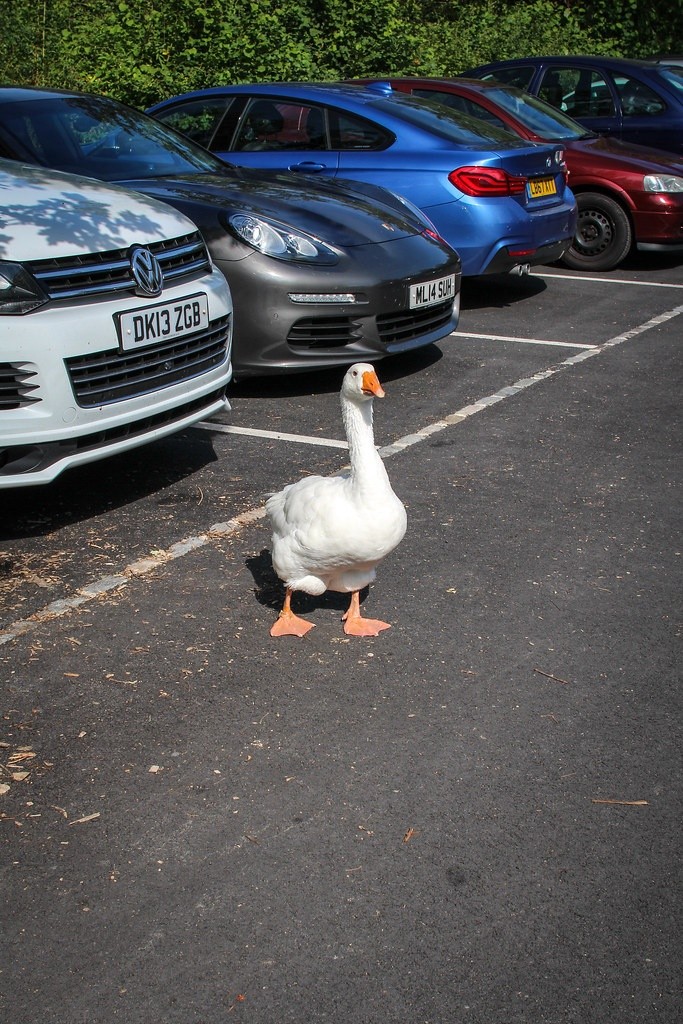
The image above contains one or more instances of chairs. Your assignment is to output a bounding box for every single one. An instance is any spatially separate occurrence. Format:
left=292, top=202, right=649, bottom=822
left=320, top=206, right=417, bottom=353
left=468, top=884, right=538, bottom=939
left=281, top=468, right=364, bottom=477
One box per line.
left=241, top=103, right=293, bottom=151
left=544, top=73, right=562, bottom=107
left=305, top=108, right=326, bottom=149
left=565, top=79, right=591, bottom=116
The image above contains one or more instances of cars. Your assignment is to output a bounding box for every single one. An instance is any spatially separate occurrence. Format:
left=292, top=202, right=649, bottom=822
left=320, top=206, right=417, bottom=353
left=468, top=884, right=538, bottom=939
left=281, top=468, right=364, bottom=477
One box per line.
left=455, top=54, right=683, bottom=156
left=0, top=153, right=236, bottom=496
left=65, top=84, right=579, bottom=290
left=561, top=58, right=683, bottom=115
left=257, top=76, right=683, bottom=271
left=0, top=85, right=464, bottom=379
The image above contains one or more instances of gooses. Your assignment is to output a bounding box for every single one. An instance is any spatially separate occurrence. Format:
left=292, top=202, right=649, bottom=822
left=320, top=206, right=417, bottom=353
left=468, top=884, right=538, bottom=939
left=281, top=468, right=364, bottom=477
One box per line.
left=262, top=363, right=409, bottom=637
